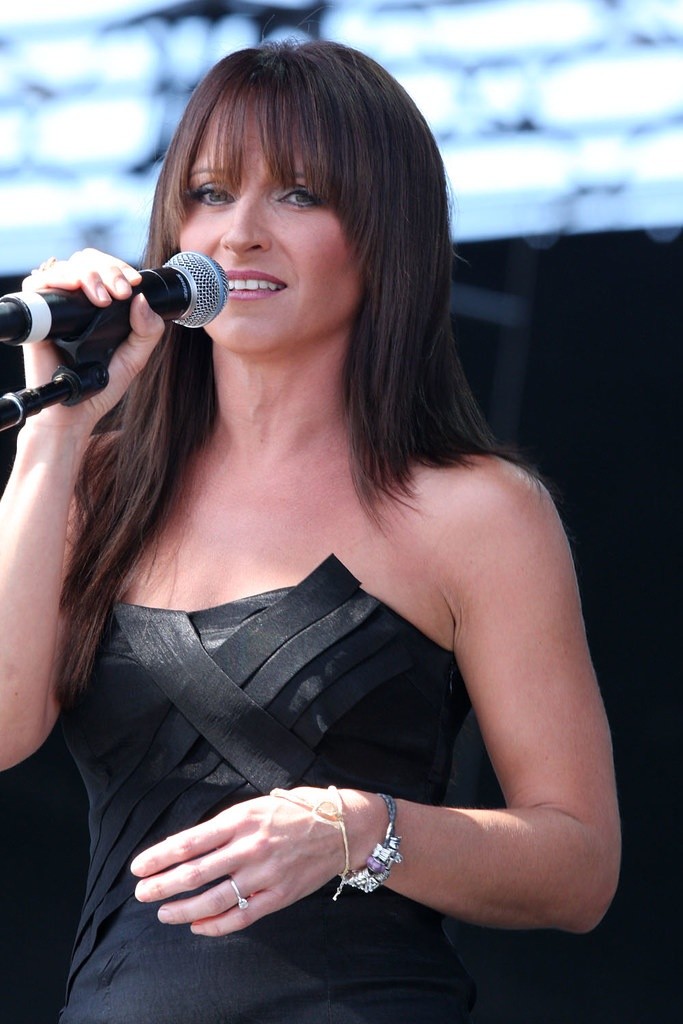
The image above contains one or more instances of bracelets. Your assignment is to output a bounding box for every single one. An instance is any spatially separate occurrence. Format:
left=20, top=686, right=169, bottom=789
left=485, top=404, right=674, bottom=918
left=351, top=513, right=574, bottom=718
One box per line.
left=270, top=784, right=350, bottom=876
left=337, top=794, right=403, bottom=892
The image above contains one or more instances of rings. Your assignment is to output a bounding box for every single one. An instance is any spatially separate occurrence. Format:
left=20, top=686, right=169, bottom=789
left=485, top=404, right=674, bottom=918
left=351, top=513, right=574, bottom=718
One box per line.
left=228, top=878, right=250, bottom=910
left=36, top=256, right=56, bottom=272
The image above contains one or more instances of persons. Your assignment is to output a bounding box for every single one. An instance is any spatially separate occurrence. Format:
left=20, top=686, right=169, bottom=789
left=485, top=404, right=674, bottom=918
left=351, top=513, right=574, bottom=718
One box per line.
left=0, top=42, right=619, bottom=1024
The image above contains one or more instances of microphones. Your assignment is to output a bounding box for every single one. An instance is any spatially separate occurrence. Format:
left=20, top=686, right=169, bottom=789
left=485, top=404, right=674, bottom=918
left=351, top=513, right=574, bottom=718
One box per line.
left=0, top=252, right=230, bottom=348
